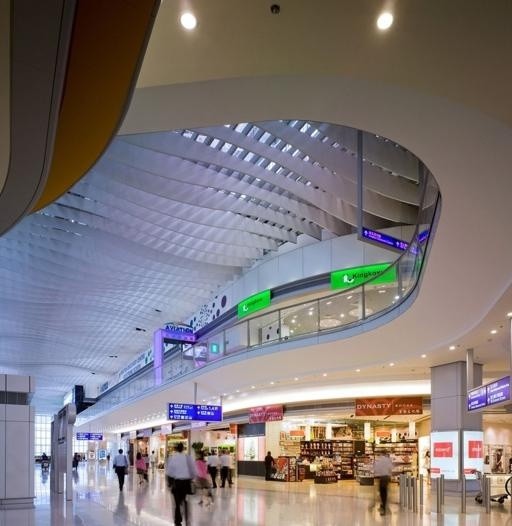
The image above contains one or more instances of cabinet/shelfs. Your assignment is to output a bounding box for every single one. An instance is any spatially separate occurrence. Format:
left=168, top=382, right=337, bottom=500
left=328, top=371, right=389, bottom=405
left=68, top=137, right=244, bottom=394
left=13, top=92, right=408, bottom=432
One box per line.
left=298, top=437, right=418, bottom=485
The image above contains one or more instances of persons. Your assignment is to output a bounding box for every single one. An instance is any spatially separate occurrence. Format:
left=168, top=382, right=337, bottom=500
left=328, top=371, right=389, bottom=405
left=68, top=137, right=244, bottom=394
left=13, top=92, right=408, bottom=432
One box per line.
left=423, top=450, right=432, bottom=486
left=264, top=450, right=276, bottom=481
left=41, top=452, right=49, bottom=470
left=112, top=449, right=156, bottom=492
left=475, top=446, right=504, bottom=504
left=372, top=448, right=394, bottom=517
left=165, top=440, right=235, bottom=526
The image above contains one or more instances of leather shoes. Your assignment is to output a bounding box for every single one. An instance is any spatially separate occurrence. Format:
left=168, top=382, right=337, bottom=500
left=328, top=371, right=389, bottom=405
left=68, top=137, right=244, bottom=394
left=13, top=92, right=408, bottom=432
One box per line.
left=212, top=482, right=235, bottom=488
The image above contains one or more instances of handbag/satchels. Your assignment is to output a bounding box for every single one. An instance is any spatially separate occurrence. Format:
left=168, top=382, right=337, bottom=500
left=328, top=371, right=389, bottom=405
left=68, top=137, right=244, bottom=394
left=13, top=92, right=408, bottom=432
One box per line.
left=189, top=482, right=197, bottom=495
